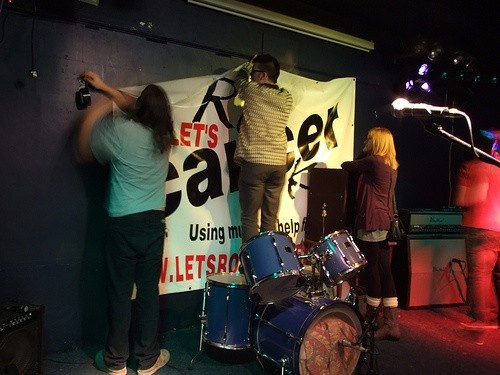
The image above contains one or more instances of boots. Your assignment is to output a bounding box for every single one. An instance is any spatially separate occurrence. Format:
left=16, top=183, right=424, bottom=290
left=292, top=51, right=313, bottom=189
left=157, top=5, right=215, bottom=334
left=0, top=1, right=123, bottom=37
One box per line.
left=367, top=307, right=400, bottom=342
left=362, top=303, right=383, bottom=330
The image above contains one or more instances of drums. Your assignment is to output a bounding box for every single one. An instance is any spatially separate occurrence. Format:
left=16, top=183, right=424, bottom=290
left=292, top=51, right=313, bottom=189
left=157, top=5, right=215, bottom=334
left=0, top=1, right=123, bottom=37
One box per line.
left=249, top=286, right=368, bottom=375
left=205, top=271, right=259, bottom=352
left=238, top=230, right=308, bottom=305
left=306, top=228, right=369, bottom=288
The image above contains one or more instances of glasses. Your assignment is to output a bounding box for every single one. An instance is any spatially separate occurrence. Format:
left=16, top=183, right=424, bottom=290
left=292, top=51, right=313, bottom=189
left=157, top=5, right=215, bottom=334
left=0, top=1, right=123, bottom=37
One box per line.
left=252, top=69, right=267, bottom=73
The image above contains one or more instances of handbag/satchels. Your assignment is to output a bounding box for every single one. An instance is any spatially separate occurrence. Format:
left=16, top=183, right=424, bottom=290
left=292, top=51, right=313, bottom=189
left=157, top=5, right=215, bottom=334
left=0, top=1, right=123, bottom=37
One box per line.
left=390, top=214, right=405, bottom=242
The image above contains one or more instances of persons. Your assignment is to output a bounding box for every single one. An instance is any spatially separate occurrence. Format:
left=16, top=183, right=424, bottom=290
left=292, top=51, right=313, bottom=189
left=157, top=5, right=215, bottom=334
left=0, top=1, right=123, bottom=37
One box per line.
left=72, top=70, right=177, bottom=375
left=454, top=128, right=500, bottom=330
left=234, top=55, right=294, bottom=274
left=340, top=127, right=400, bottom=339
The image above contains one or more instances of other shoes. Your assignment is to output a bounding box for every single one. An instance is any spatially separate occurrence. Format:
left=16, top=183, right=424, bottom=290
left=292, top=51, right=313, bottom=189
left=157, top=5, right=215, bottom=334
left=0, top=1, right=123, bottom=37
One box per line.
left=137, top=349, right=171, bottom=375
left=96, top=349, right=127, bottom=375
left=460, top=318, right=499, bottom=329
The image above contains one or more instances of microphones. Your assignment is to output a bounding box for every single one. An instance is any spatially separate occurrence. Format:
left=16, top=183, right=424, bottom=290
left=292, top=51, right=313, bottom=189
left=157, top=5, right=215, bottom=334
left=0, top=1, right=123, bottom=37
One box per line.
left=452, top=258, right=464, bottom=263
left=338, top=338, right=368, bottom=353
left=390, top=98, right=465, bottom=120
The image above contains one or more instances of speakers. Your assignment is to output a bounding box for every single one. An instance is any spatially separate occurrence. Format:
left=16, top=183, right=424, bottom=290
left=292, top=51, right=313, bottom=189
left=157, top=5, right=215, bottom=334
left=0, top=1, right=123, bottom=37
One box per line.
left=391, top=232, right=469, bottom=310
left=0, top=306, right=43, bottom=375
